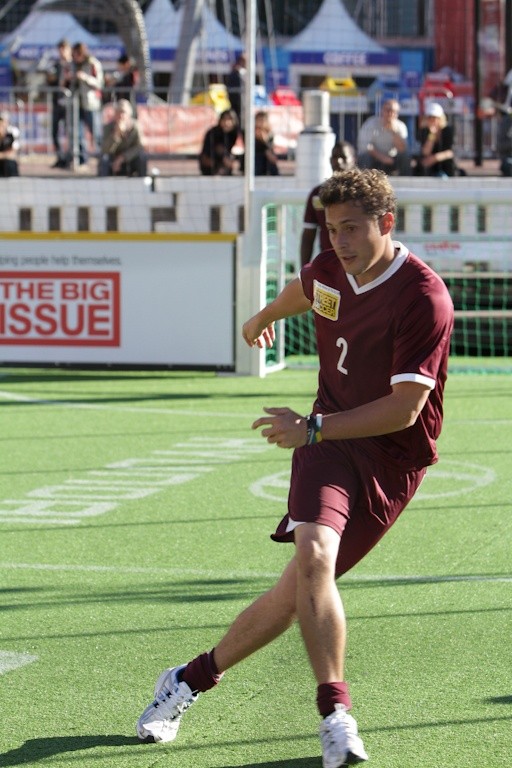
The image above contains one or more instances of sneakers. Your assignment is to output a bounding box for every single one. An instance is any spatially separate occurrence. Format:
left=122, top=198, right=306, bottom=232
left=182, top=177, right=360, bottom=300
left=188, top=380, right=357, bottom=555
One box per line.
left=135, top=663, right=199, bottom=743
left=317, top=704, right=369, bottom=768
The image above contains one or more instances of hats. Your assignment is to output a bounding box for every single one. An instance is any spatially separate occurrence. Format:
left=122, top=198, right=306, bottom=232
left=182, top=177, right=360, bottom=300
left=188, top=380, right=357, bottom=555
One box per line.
left=425, top=103, right=444, bottom=117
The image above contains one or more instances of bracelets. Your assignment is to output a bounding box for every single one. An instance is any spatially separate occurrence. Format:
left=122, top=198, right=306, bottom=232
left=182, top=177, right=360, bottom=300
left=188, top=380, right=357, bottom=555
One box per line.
left=304, top=412, right=323, bottom=445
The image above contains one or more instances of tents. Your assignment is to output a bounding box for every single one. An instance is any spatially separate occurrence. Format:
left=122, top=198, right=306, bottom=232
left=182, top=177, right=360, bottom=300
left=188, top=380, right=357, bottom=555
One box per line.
left=141, top=0, right=243, bottom=77
left=0, top=0, right=127, bottom=80
left=276, top=0, right=399, bottom=93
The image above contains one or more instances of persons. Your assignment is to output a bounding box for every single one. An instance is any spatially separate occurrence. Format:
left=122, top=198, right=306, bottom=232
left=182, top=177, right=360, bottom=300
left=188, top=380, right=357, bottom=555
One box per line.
left=137, top=164, right=457, bottom=768
left=355, top=98, right=410, bottom=177
left=0, top=110, right=23, bottom=176
left=198, top=110, right=298, bottom=176
left=96, top=98, right=146, bottom=178
left=409, top=101, right=455, bottom=175
left=301, top=140, right=358, bottom=271
left=43, top=40, right=104, bottom=168
